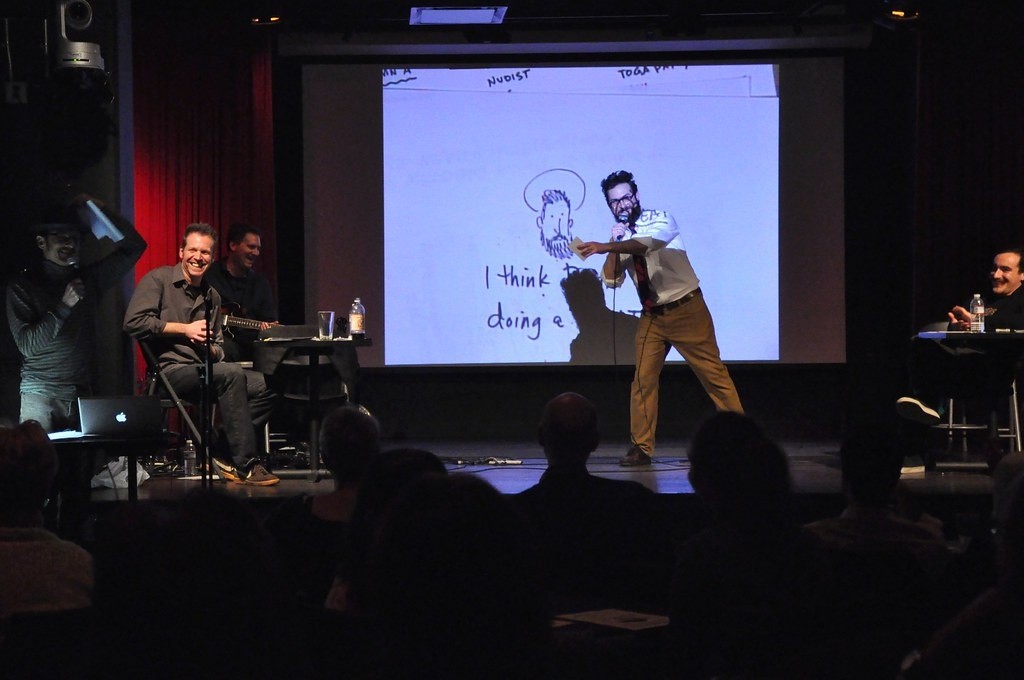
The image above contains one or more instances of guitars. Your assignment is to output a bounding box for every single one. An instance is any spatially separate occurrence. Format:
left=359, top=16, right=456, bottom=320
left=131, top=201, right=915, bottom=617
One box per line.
left=221, top=303, right=289, bottom=340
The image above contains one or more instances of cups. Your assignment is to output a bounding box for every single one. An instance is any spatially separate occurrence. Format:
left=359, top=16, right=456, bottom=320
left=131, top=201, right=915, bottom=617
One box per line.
left=318, top=311, right=335, bottom=341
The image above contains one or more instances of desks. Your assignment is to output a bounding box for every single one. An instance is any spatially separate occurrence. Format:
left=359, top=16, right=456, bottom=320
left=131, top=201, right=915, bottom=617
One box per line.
left=918, top=331, right=1024, bottom=472
left=254, top=337, right=372, bottom=483
left=48, top=436, right=156, bottom=528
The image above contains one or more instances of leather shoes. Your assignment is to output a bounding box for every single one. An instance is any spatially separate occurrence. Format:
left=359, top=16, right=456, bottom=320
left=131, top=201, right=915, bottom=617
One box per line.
left=620, top=446, right=652, bottom=465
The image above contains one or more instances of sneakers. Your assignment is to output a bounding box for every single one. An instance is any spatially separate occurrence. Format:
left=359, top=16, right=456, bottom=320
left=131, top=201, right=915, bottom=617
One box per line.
left=245, top=463, right=279, bottom=485
left=212, top=456, right=241, bottom=481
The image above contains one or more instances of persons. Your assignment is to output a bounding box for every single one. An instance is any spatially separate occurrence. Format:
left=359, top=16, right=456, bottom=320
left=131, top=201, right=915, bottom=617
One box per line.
left=893, top=241, right=1024, bottom=426
left=5, top=194, right=145, bottom=430
left=122, top=221, right=288, bottom=488
left=0, top=391, right=1024, bottom=680
left=577, top=169, right=748, bottom=466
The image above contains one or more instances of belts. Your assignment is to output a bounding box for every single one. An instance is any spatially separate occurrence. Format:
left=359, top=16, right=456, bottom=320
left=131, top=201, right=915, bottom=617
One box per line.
left=643, top=287, right=701, bottom=312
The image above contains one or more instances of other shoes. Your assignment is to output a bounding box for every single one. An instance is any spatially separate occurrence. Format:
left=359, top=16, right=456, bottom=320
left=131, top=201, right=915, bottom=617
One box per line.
left=898, top=457, right=926, bottom=474
left=895, top=396, right=942, bottom=428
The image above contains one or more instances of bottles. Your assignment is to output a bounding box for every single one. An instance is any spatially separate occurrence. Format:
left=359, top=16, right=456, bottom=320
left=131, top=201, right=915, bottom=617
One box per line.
left=970, top=293, right=985, bottom=333
left=349, top=298, right=365, bottom=339
left=183, top=440, right=196, bottom=475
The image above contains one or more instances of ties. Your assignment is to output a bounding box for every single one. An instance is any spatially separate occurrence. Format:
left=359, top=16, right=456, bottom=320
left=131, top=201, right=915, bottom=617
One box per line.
left=627, top=221, right=659, bottom=307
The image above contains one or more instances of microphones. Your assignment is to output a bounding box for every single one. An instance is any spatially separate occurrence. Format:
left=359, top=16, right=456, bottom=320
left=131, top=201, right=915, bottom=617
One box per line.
left=67, top=256, right=81, bottom=281
left=616, top=213, right=629, bottom=243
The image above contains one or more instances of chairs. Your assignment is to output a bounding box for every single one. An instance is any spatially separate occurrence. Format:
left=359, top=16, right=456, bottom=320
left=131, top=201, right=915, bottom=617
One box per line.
left=138, top=339, right=228, bottom=484
left=916, top=322, right=1016, bottom=457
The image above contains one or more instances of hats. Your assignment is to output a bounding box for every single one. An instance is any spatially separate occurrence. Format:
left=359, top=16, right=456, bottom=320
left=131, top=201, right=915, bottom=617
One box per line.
left=28, top=202, right=91, bottom=235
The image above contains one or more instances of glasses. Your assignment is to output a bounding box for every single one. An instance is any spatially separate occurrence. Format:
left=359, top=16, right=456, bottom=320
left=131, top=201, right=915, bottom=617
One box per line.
left=44, top=229, right=82, bottom=246
left=986, top=520, right=1009, bottom=536
left=609, top=191, right=635, bottom=209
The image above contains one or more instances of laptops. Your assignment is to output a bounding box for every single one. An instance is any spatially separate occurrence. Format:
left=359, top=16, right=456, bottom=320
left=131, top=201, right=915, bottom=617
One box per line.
left=77, top=396, right=163, bottom=436
